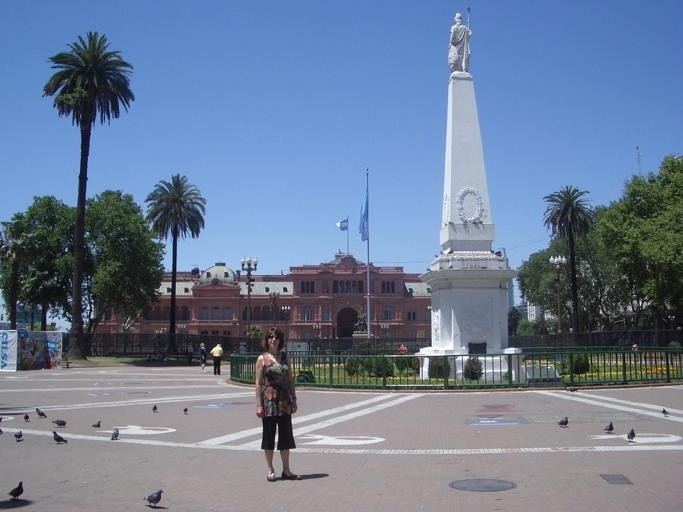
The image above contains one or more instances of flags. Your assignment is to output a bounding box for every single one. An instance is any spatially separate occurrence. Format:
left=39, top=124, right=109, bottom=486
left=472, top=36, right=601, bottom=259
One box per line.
left=335, top=216, right=347, bottom=231
left=358, top=180, right=368, bottom=241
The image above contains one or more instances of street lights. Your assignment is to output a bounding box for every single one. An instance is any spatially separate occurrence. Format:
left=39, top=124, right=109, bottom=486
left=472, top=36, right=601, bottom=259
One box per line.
left=269, top=288, right=280, bottom=324
left=312, top=324, right=322, bottom=337
left=240, top=256, right=259, bottom=337
left=381, top=324, right=390, bottom=346
left=549, top=256, right=566, bottom=333
left=281, top=305, right=291, bottom=353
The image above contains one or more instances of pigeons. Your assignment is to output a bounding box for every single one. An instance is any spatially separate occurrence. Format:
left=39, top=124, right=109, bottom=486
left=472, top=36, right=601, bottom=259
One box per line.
left=661, top=407, right=670, bottom=417
left=603, top=422, right=614, bottom=433
left=14, top=407, right=68, bottom=444
left=92, top=420, right=101, bottom=427
left=626, top=428, right=636, bottom=441
left=142, top=487, right=164, bottom=507
left=183, top=408, right=189, bottom=415
left=111, top=427, right=120, bottom=440
left=6, top=480, right=24, bottom=500
left=556, top=416, right=569, bottom=429
left=152, top=404, right=157, bottom=412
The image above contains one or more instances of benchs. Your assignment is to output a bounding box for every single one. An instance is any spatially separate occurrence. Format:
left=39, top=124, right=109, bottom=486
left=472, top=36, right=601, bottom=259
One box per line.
left=61, top=361, right=72, bottom=368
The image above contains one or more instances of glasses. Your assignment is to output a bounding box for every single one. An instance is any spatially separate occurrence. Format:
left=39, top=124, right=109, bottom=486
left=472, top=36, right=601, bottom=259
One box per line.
left=269, top=335, right=279, bottom=339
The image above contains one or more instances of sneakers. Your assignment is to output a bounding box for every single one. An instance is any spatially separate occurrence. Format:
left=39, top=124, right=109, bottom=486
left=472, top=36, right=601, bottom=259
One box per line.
left=281, top=471, right=303, bottom=480
left=213, top=372, right=219, bottom=374
left=267, top=471, right=276, bottom=480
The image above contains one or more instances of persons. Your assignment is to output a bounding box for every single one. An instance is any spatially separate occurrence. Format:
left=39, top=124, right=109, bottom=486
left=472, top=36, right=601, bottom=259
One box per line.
left=210, top=343, right=223, bottom=374
left=187, top=341, right=194, bottom=365
left=199, top=342, right=206, bottom=372
left=632, top=341, right=638, bottom=361
left=447, top=12, right=472, bottom=72
left=255, top=326, right=300, bottom=481
